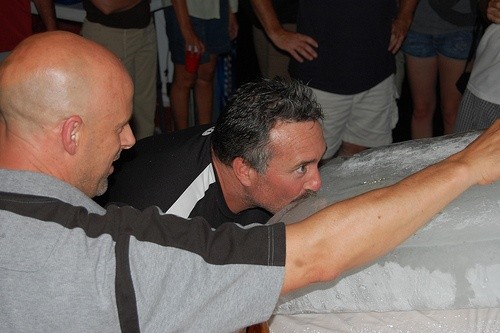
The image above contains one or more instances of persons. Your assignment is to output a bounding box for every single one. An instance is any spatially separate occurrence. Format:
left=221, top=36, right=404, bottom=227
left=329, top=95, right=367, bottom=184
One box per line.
left=454, top=0, right=500, bottom=136
left=161, top=0, right=240, bottom=137
left=92, top=72, right=327, bottom=230
left=250, top=0, right=420, bottom=164
left=244, top=0, right=299, bottom=81
left=399, top=0, right=477, bottom=139
left=0, top=0, right=57, bottom=63
left=80, top=0, right=158, bottom=140
left=0, top=30, right=500, bottom=333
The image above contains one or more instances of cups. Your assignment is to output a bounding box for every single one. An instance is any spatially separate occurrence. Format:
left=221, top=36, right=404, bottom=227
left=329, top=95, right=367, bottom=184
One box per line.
left=185, top=45, right=201, bottom=71
